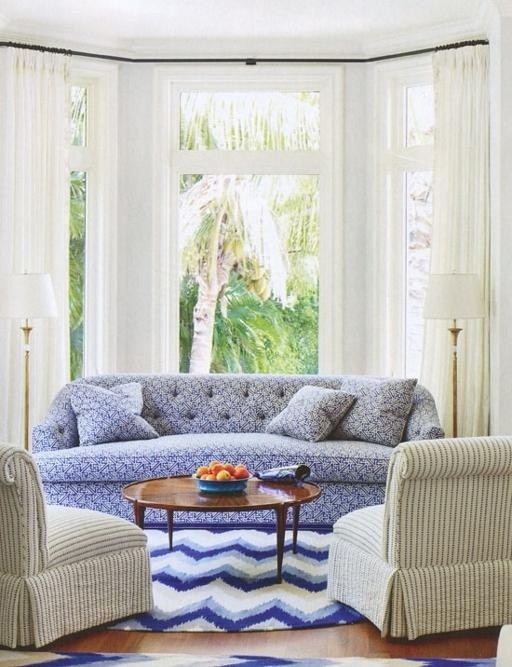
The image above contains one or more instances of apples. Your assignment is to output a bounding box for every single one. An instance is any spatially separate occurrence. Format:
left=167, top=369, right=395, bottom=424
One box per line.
left=196, top=461, right=250, bottom=480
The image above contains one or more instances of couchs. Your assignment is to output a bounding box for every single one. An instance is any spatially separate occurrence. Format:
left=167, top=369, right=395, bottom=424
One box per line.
left=29, top=372, right=445, bottom=525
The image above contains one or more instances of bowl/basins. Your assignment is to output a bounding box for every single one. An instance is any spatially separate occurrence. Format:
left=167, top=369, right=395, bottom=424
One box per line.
left=192, top=472, right=252, bottom=494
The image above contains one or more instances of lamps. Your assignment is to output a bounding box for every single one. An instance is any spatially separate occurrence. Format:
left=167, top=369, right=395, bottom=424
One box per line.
left=425, top=271, right=486, bottom=435
left=9, top=268, right=59, bottom=449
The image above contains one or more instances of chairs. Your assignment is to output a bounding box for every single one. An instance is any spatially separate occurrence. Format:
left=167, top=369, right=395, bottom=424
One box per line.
left=0, top=440, right=157, bottom=648
left=323, top=432, right=512, bottom=642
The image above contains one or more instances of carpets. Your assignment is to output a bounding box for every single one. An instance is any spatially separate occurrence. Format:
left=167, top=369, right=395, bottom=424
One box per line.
left=97, top=515, right=370, bottom=633
left=1, top=652, right=500, bottom=665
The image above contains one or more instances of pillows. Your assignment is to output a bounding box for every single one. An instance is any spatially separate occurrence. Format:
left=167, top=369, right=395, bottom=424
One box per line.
left=333, top=376, right=416, bottom=447
left=264, top=382, right=358, bottom=441
left=61, top=380, right=160, bottom=446
